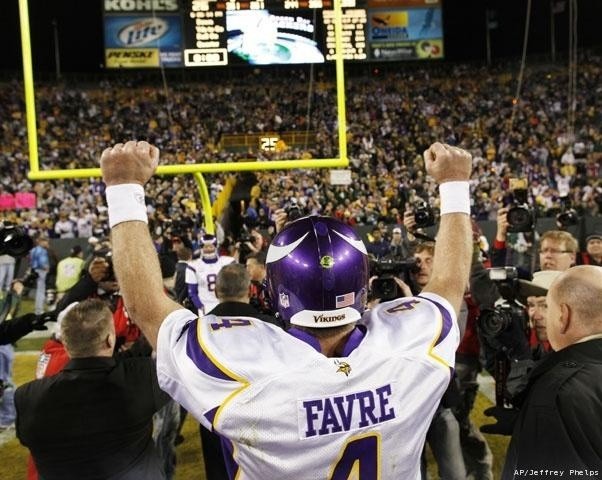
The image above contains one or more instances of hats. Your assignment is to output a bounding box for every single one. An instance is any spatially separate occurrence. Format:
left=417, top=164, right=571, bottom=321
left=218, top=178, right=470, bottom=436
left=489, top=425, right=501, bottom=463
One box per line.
left=392, top=227, right=402, bottom=234
left=70, top=245, right=82, bottom=257
left=512, top=270, right=565, bottom=299
left=584, top=230, right=602, bottom=242
left=87, top=235, right=99, bottom=244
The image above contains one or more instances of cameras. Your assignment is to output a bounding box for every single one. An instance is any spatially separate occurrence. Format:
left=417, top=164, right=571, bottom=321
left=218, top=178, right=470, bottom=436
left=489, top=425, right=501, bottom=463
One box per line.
left=506, top=207, right=533, bottom=231
left=0, top=220, right=35, bottom=258
left=409, top=201, right=440, bottom=227
left=100, top=255, right=115, bottom=281
left=558, top=211, right=577, bottom=226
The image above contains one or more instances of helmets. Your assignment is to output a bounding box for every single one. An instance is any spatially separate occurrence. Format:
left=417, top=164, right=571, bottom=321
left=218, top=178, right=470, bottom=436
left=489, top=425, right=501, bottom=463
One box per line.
left=263, top=214, right=370, bottom=329
left=199, top=234, right=219, bottom=260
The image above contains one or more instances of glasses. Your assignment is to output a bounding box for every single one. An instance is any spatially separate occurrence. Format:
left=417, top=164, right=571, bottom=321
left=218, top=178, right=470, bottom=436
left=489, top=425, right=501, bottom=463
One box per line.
left=538, top=246, right=573, bottom=256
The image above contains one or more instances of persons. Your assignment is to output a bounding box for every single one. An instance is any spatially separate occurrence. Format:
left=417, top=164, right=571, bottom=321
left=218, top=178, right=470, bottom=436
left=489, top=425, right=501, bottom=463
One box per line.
left=0, top=71, right=200, bottom=479
left=98, top=138, right=478, bottom=480
left=424, top=48, right=599, bottom=479
left=200, top=60, right=425, bottom=314
